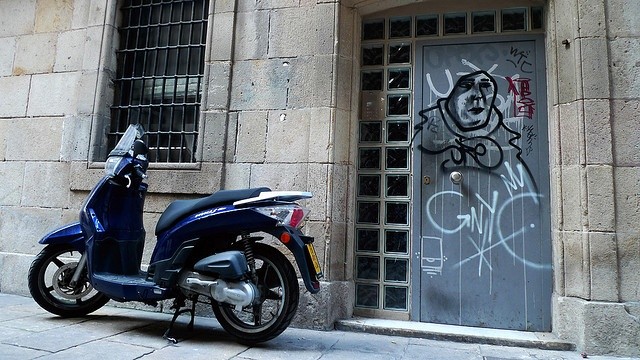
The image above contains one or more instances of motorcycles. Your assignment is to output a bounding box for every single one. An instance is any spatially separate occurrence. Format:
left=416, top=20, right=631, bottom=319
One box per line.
left=28, top=123, right=323, bottom=345
